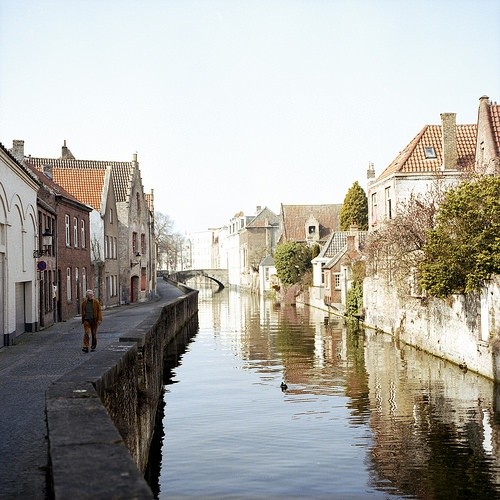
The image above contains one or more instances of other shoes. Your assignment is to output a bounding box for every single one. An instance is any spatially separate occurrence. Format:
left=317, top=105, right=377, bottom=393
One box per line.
left=82, top=347, right=89, bottom=352
left=91, top=345, right=96, bottom=350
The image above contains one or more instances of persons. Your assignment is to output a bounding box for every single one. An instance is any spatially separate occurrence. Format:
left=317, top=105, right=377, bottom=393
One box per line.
left=81, top=290, right=103, bottom=352
